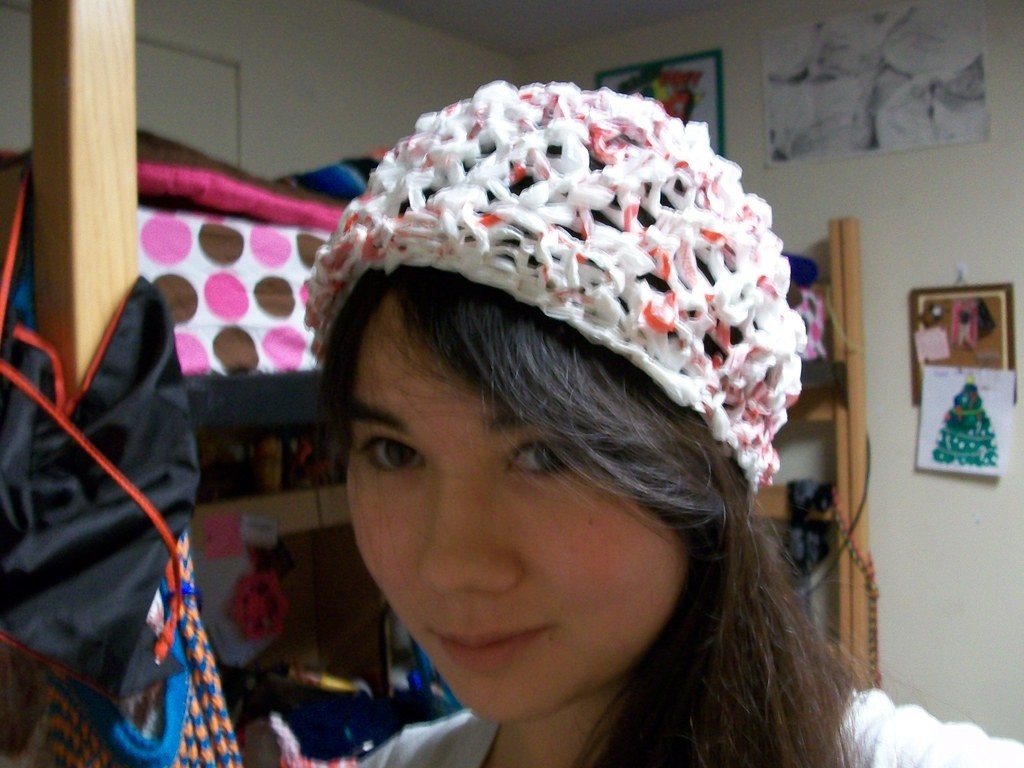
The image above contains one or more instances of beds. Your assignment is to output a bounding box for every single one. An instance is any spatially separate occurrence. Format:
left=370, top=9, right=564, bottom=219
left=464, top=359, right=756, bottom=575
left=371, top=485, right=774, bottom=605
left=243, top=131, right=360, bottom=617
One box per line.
left=0, top=0, right=867, bottom=429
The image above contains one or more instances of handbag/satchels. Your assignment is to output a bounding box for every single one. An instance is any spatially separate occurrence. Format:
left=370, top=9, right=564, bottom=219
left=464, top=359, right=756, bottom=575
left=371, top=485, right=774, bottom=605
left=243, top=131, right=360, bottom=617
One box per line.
left=40, top=529, right=244, bottom=768
left=0, top=359, right=244, bottom=768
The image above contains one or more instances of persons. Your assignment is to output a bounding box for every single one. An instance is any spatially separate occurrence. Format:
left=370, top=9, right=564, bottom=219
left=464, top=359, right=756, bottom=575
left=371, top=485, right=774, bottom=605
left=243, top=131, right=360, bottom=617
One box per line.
left=305, top=78, right=1024, bottom=768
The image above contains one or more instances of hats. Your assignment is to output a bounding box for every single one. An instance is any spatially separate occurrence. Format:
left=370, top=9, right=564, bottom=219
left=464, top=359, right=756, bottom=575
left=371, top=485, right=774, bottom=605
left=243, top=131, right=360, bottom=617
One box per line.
left=304, top=81, right=808, bottom=494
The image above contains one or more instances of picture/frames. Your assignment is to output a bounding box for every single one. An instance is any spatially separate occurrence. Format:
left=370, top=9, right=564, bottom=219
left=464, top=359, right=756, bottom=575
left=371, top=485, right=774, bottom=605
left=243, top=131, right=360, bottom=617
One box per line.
left=908, top=283, right=1014, bottom=408
left=594, top=48, right=724, bottom=159
